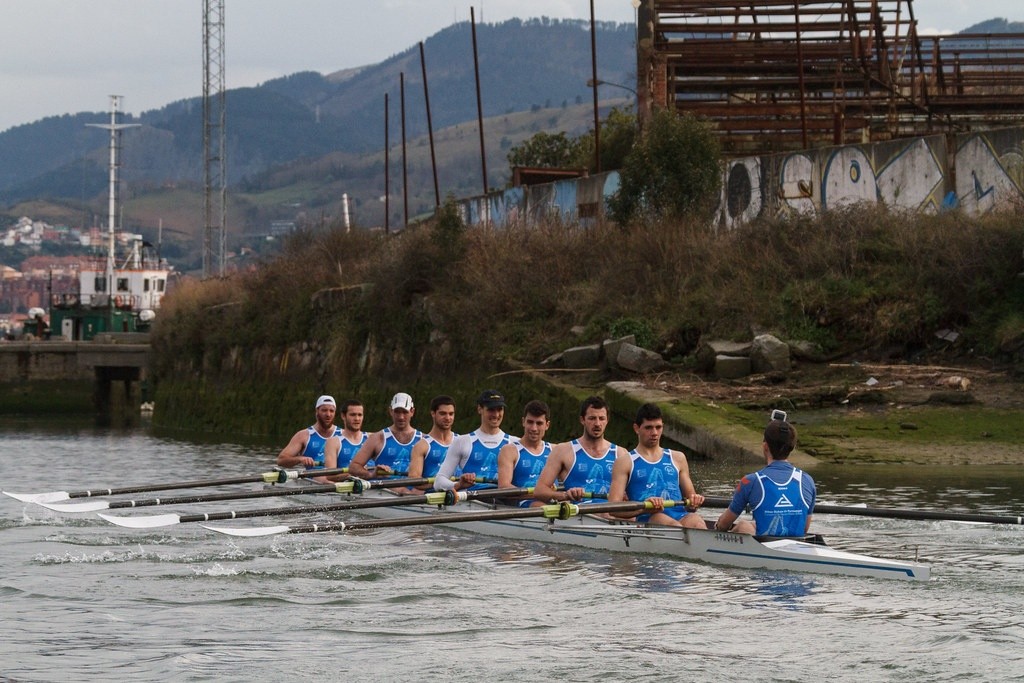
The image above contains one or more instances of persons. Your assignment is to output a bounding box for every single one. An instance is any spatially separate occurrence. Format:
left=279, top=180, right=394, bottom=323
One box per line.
left=608, top=403, right=708, bottom=529
left=325, top=399, right=375, bottom=482
left=277, top=395, right=345, bottom=482
left=434, top=389, right=522, bottom=492
left=498, top=400, right=558, bottom=508
left=408, top=395, right=460, bottom=493
left=534, top=397, right=636, bottom=522
left=348, top=393, right=428, bottom=496
left=714, top=420, right=816, bottom=536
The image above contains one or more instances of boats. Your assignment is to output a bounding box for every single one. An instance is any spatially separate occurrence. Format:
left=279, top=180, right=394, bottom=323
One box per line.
left=261, top=465, right=937, bottom=587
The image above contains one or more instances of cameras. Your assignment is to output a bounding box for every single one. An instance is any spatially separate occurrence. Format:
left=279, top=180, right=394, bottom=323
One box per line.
left=771, top=409, right=786, bottom=424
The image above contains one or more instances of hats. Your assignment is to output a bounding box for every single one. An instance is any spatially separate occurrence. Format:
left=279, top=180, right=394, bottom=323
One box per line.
left=391, top=393, right=413, bottom=411
left=478, top=390, right=507, bottom=409
left=316, top=395, right=336, bottom=408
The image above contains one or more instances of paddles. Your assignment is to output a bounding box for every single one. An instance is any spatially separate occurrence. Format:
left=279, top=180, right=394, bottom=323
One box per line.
left=1, top=463, right=377, bottom=504
left=581, top=492, right=610, bottom=499
left=683, top=496, right=1024, bottom=527
left=197, top=498, right=692, bottom=539
left=92, top=482, right=564, bottom=519
left=36, top=474, right=461, bottom=514
left=449, top=475, right=498, bottom=485
left=377, top=469, right=409, bottom=476
